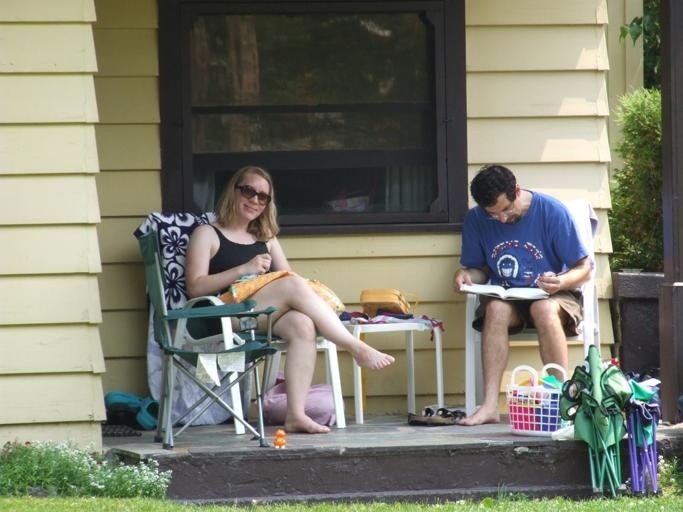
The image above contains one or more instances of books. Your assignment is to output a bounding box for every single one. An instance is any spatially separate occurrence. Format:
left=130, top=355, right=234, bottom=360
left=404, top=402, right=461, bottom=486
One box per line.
left=459, top=282, right=551, bottom=301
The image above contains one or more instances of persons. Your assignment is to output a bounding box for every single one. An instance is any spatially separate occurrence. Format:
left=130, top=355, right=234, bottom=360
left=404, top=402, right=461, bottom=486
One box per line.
left=183, top=164, right=394, bottom=432
left=452, top=163, right=594, bottom=427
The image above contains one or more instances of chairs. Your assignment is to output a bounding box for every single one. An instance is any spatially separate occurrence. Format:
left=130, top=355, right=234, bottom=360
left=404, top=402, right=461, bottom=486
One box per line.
left=463, top=201, right=600, bottom=419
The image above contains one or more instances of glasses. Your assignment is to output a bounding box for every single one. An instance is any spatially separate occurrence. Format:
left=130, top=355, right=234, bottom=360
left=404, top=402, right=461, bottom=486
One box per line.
left=236, top=185, right=271, bottom=205
left=482, top=201, right=515, bottom=221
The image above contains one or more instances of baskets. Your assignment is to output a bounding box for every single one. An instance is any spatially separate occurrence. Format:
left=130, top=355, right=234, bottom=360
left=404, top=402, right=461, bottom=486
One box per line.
left=506, top=363, right=573, bottom=437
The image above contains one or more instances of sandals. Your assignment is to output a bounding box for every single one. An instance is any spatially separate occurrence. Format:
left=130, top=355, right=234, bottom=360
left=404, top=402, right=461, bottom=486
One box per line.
left=104, top=391, right=159, bottom=430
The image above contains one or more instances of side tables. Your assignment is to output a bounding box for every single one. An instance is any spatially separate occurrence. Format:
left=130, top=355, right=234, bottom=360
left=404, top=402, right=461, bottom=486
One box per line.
left=341, top=316, right=445, bottom=425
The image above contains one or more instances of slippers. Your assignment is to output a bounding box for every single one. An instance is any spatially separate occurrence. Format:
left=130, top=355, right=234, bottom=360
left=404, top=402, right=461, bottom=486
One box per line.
left=409, top=408, right=465, bottom=426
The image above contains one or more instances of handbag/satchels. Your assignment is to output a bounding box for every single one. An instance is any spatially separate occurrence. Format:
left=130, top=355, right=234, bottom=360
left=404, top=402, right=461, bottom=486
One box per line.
left=360, top=287, right=420, bottom=316
